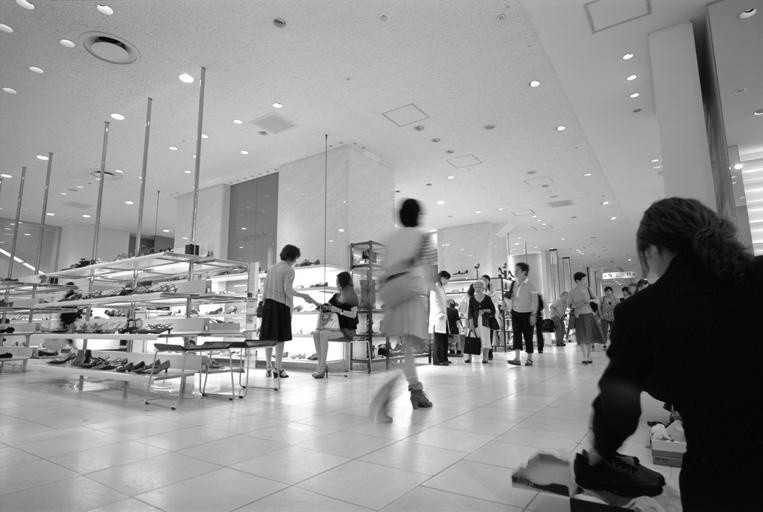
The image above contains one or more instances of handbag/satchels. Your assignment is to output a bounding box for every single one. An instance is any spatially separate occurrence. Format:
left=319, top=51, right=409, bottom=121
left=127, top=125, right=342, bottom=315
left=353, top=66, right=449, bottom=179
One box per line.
left=500, top=292, right=512, bottom=312
left=590, top=297, right=597, bottom=312
left=378, top=257, right=427, bottom=310
left=316, top=312, right=340, bottom=332
left=464, top=330, right=481, bottom=355
left=256, top=301, right=264, bottom=317
left=482, top=312, right=499, bottom=330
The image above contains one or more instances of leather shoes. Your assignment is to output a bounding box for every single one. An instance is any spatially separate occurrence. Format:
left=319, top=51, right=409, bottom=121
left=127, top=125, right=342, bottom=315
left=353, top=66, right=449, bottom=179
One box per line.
left=272, top=352, right=318, bottom=360
left=294, top=259, right=320, bottom=267
left=209, top=306, right=237, bottom=314
left=38, top=351, right=170, bottom=374
left=310, top=282, right=328, bottom=288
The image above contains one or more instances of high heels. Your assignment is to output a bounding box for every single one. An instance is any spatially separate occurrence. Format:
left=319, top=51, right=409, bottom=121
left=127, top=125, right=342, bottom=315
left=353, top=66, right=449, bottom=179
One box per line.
left=408, top=383, right=432, bottom=409
left=266, top=366, right=288, bottom=378
left=312, top=365, right=328, bottom=378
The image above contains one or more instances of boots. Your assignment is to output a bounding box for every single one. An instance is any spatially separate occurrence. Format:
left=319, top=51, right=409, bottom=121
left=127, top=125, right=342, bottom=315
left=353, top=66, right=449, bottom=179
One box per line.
left=358, top=280, right=375, bottom=309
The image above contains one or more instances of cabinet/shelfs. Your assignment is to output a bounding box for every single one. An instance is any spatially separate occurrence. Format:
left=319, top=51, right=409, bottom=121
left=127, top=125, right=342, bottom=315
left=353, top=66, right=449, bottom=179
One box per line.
left=0, top=251, right=519, bottom=411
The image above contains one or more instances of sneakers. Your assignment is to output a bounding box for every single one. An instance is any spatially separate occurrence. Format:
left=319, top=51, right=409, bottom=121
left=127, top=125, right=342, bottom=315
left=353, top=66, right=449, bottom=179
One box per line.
left=557, top=343, right=565, bottom=346
left=581, top=345, right=606, bottom=365
left=574, top=449, right=665, bottom=498
left=433, top=361, right=451, bottom=365
left=539, top=348, right=543, bottom=353
left=508, top=358, right=533, bottom=366
left=465, top=350, right=493, bottom=363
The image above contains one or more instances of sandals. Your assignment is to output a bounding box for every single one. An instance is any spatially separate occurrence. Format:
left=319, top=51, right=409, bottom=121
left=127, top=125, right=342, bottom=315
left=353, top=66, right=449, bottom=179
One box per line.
left=118, top=319, right=173, bottom=334
left=58, top=284, right=177, bottom=302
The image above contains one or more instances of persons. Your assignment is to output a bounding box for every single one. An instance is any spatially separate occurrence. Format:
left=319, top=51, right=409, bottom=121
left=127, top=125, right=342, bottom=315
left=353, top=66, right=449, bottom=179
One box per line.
left=313, top=272, right=358, bottom=378
left=60, top=283, right=77, bottom=346
left=428, top=263, right=648, bottom=366
left=258, top=244, right=310, bottom=377
left=589, top=198, right=763, bottom=512
left=367, top=199, right=437, bottom=422
